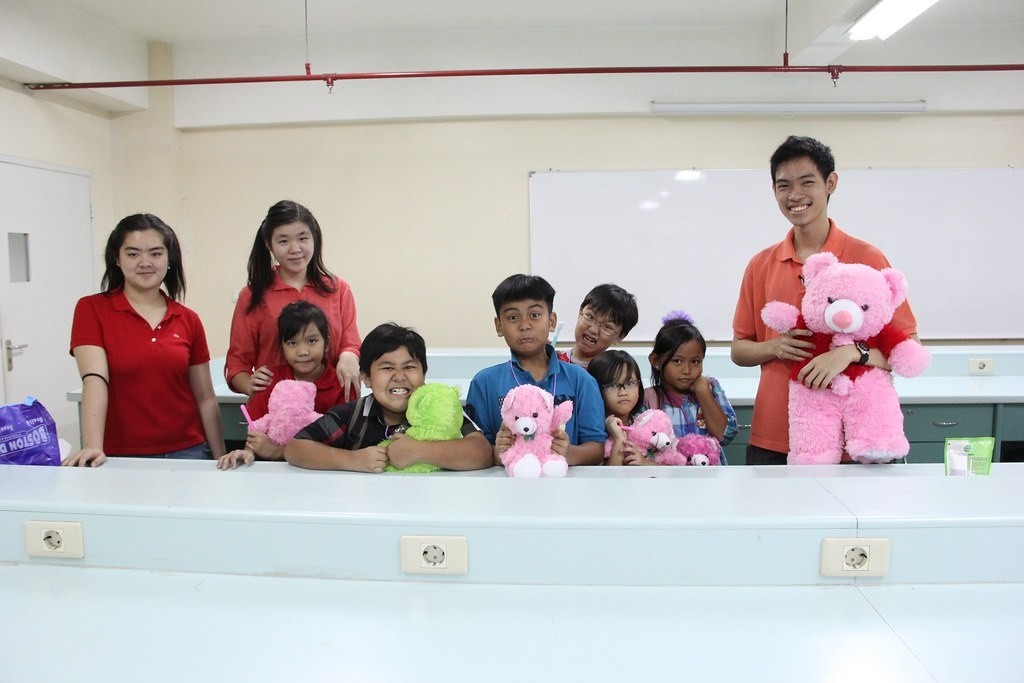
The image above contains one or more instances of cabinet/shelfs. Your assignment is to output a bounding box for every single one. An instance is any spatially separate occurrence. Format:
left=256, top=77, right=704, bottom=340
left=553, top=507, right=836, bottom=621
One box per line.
left=219, top=402, right=249, bottom=442
left=720, top=405, right=754, bottom=465
left=892, top=403, right=1004, bottom=463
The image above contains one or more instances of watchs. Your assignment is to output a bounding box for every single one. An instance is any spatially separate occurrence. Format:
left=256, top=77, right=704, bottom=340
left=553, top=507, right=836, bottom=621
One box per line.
left=856, top=340, right=870, bottom=366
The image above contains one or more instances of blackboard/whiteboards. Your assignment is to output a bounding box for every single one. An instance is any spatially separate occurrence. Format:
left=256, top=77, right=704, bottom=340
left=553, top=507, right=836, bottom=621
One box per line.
left=527, top=169, right=1023, bottom=347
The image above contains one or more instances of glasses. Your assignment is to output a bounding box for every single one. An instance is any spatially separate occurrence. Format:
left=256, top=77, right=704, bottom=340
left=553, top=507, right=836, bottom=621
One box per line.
left=602, top=379, right=640, bottom=393
left=580, top=312, right=623, bottom=337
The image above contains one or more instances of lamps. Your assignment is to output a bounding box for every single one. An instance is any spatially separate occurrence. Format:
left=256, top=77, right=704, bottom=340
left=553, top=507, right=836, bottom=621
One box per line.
left=648, top=100, right=928, bottom=116
left=837, top=0, right=941, bottom=42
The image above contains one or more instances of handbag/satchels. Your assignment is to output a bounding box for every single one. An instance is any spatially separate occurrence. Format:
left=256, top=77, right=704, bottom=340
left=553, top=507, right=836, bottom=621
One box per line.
left=1, top=395, right=61, bottom=466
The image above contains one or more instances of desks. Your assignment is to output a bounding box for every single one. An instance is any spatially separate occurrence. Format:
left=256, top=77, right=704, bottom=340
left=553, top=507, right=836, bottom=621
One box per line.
left=58, top=372, right=1024, bottom=404
left=0, top=561, right=1024, bottom=683
left=0, top=455, right=1024, bottom=520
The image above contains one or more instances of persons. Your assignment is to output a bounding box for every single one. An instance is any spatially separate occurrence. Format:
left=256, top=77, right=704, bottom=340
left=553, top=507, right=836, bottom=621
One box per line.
left=731, top=134, right=922, bottom=465
left=216, top=200, right=739, bottom=473
left=60, top=214, right=227, bottom=468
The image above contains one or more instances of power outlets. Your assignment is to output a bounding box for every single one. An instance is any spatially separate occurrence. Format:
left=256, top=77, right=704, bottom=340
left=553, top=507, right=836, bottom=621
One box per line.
left=968, top=357, right=998, bottom=374
left=399, top=535, right=469, bottom=575
left=24, top=521, right=85, bottom=560
left=819, top=537, right=890, bottom=578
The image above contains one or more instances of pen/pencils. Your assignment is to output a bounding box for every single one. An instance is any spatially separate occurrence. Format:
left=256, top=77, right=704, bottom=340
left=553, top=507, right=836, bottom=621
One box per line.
left=550, top=322, right=563, bottom=348
left=798, top=275, right=804, bottom=284
left=241, top=404, right=255, bottom=431
left=619, top=425, right=632, bottom=431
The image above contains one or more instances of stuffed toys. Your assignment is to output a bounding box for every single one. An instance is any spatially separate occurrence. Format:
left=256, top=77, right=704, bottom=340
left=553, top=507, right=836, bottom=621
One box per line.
left=603, top=407, right=686, bottom=466
left=248, top=379, right=326, bottom=446
left=673, top=431, right=721, bottom=467
left=500, top=384, right=574, bottom=476
left=379, top=384, right=464, bottom=473
left=759, top=252, right=930, bottom=465
